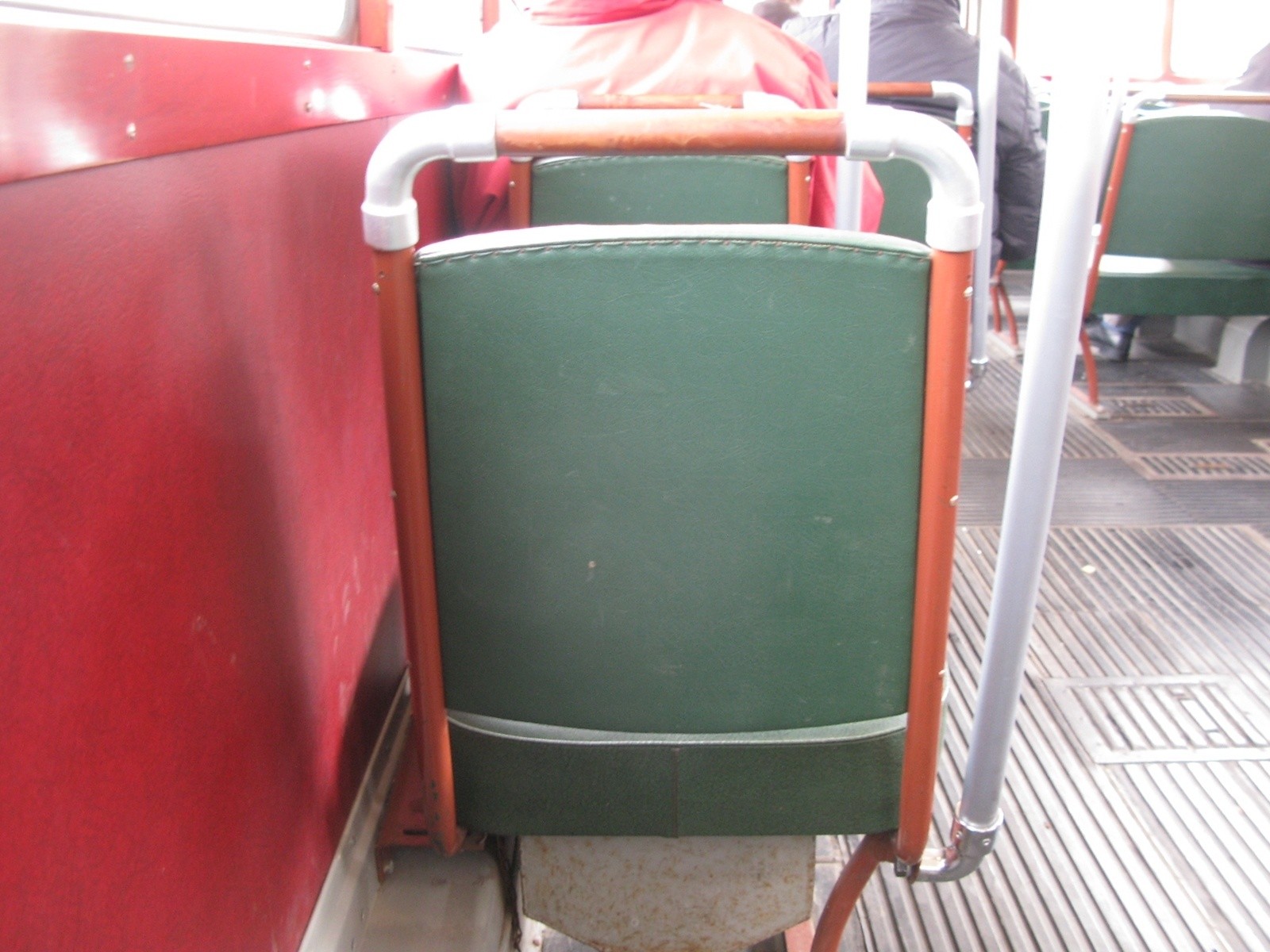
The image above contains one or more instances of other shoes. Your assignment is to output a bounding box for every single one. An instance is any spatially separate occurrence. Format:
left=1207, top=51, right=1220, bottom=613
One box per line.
left=1084, top=317, right=1134, bottom=363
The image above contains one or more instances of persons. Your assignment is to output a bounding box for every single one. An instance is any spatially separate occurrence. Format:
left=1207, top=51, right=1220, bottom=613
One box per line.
left=780, top=0, right=1044, bottom=277
left=446, top=0, right=884, bottom=237
left=1082, top=42, right=1270, bottom=363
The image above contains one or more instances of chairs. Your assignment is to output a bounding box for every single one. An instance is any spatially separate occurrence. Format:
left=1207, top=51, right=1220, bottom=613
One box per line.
left=361, top=78, right=1270, bottom=952
